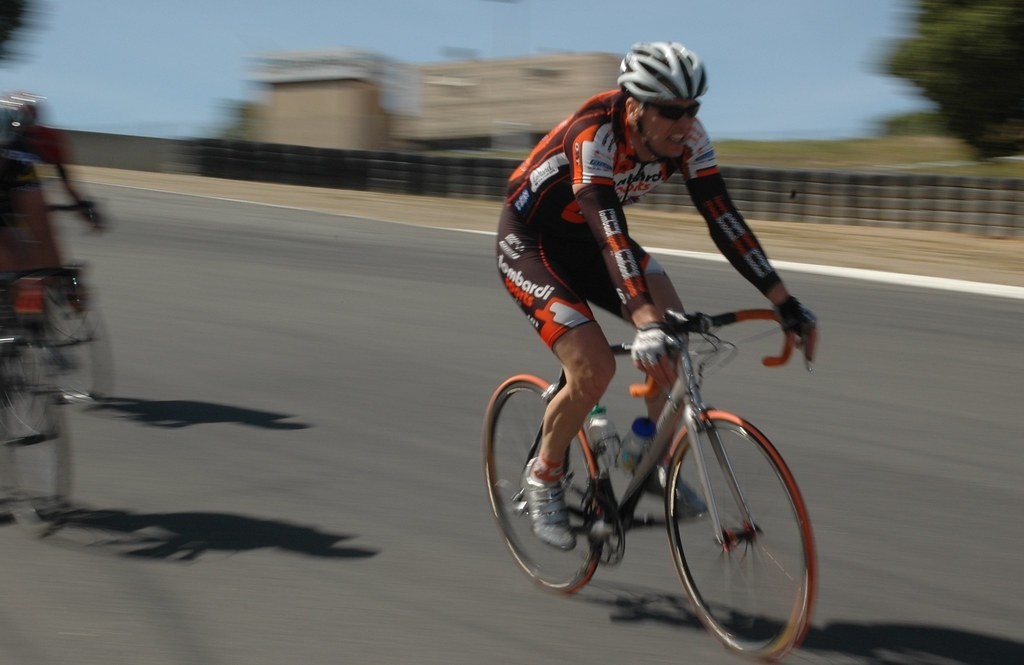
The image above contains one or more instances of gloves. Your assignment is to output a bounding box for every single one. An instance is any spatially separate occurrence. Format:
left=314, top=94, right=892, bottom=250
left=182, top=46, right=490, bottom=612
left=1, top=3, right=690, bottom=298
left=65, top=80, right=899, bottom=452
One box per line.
left=631, top=322, right=679, bottom=389
left=778, top=296, right=816, bottom=360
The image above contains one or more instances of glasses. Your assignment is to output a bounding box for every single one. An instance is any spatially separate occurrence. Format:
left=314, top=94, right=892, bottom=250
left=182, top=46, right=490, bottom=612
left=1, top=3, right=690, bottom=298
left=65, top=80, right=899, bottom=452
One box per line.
left=648, top=101, right=699, bottom=120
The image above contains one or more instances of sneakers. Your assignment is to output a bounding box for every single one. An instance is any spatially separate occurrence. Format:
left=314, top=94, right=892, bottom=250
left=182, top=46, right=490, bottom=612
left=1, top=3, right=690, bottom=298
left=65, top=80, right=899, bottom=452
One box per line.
left=675, top=472, right=706, bottom=511
left=522, top=458, right=576, bottom=551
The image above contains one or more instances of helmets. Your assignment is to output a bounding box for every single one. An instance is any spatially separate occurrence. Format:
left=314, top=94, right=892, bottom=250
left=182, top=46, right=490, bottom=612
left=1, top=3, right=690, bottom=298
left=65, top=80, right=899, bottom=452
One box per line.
left=617, top=41, right=708, bottom=101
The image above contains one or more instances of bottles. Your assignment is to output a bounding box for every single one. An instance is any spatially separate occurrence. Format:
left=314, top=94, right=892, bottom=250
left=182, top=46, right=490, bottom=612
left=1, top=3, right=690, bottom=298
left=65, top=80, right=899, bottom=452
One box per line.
left=618, top=416, right=656, bottom=475
left=587, top=404, right=620, bottom=473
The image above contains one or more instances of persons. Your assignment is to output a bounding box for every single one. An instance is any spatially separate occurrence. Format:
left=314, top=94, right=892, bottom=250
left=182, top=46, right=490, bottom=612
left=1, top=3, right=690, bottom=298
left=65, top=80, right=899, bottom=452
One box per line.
left=496, top=42, right=816, bottom=549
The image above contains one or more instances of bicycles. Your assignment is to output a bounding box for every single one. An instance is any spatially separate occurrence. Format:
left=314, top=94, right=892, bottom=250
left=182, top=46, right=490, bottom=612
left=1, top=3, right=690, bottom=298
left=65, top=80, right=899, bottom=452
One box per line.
left=0, top=196, right=105, bottom=537
left=482, top=305, right=817, bottom=665
